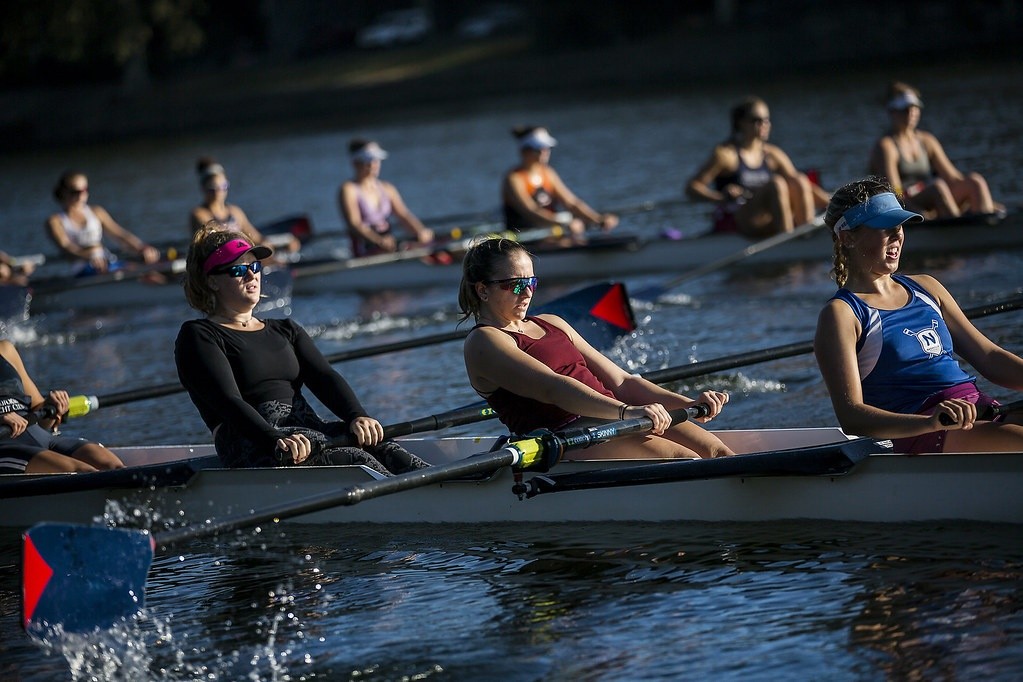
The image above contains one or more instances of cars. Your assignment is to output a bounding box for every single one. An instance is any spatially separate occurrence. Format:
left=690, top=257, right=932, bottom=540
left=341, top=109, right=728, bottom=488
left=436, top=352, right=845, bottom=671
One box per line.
left=460, top=7, right=524, bottom=41
left=356, top=9, right=429, bottom=51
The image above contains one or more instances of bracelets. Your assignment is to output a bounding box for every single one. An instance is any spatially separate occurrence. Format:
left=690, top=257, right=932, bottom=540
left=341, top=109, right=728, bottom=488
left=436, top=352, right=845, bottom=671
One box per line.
left=618, top=403, right=628, bottom=421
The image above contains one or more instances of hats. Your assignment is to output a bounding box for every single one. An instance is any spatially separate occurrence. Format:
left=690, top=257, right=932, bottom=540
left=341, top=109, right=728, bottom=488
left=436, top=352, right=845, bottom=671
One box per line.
left=203, top=238, right=273, bottom=272
left=353, top=142, right=389, bottom=164
left=519, top=130, right=558, bottom=150
left=833, top=193, right=924, bottom=240
left=887, top=92, right=924, bottom=110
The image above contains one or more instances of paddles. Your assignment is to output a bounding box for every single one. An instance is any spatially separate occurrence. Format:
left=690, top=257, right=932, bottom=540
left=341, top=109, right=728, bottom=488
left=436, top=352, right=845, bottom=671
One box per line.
left=19, top=402, right=713, bottom=639
left=938, top=398, right=1023, bottom=427
left=265, top=221, right=598, bottom=287
left=272, top=296, right=1022, bottom=464
left=631, top=181, right=927, bottom=305
left=1, top=277, right=640, bottom=440
left=31, top=213, right=314, bottom=280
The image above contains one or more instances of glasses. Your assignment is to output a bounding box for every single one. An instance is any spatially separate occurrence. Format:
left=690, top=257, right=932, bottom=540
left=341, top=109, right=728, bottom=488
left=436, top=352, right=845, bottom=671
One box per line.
left=208, top=260, right=261, bottom=277
left=752, top=115, right=769, bottom=124
left=71, top=188, right=92, bottom=197
left=210, top=183, right=230, bottom=194
left=473, top=276, right=539, bottom=295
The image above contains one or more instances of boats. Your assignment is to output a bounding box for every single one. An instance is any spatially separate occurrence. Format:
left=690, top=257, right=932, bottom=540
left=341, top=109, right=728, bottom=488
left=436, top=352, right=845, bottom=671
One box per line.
left=0, top=428, right=1022, bottom=532
left=0, top=205, right=1023, bottom=315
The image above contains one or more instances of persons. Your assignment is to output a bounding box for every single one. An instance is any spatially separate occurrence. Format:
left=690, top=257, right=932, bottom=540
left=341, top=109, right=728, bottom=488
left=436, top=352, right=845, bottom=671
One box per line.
left=814, top=178, right=1023, bottom=452
left=172, top=229, right=433, bottom=476
left=502, top=125, right=618, bottom=248
left=339, top=138, right=432, bottom=255
left=44, top=169, right=166, bottom=286
left=870, top=82, right=1004, bottom=220
left=684, top=99, right=829, bottom=235
left=0, top=338, right=126, bottom=473
left=189, top=157, right=299, bottom=262
left=458, top=239, right=736, bottom=459
